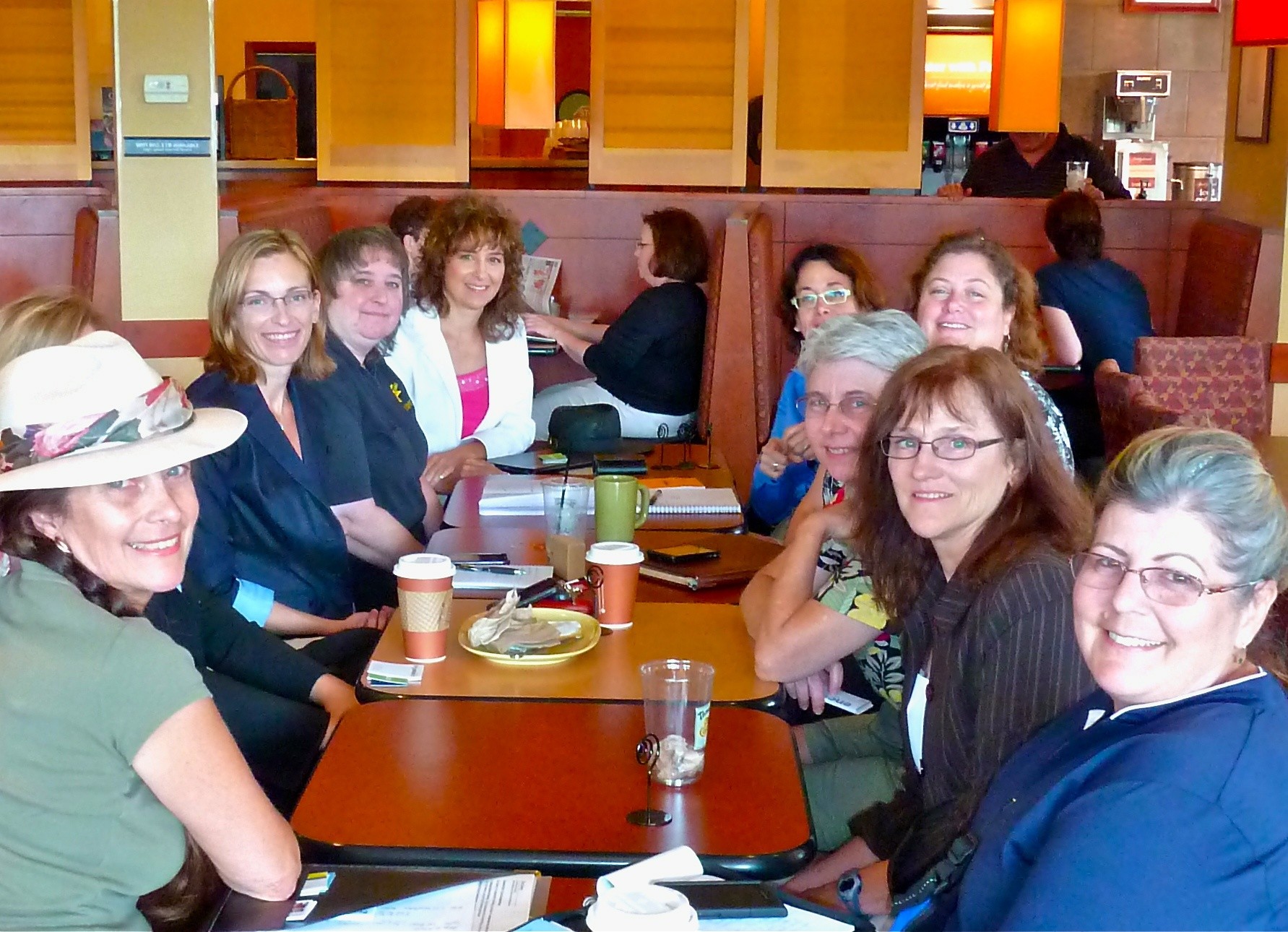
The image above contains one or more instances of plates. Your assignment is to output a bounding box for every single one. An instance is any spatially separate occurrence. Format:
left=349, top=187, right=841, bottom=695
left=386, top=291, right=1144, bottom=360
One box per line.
left=585, top=902, right=698, bottom=932
left=455, top=607, right=599, bottom=666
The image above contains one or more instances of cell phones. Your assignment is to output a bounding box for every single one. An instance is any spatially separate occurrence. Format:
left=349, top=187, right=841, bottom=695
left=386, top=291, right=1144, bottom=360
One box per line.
left=441, top=552, right=510, bottom=566
left=650, top=879, right=788, bottom=920
left=646, top=544, right=722, bottom=565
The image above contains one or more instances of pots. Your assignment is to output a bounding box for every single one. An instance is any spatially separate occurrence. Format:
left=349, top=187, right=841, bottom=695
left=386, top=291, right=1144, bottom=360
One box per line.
left=1171, top=162, right=1221, bottom=203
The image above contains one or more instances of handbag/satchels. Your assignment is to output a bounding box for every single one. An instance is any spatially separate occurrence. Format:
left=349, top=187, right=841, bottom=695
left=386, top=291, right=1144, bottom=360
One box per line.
left=223, top=65, right=297, bottom=159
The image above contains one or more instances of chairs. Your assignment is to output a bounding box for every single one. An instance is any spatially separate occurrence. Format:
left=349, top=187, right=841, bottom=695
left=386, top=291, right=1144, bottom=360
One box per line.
left=1133, top=330, right=1266, bottom=440
left=1095, top=356, right=1178, bottom=468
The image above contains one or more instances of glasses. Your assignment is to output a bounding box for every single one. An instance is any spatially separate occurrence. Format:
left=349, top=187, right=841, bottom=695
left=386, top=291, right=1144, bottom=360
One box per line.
left=1067, top=547, right=1270, bottom=607
left=795, top=395, right=879, bottom=418
left=635, top=240, right=653, bottom=250
left=790, top=289, right=856, bottom=310
left=880, top=436, right=1006, bottom=461
left=236, top=290, right=316, bottom=312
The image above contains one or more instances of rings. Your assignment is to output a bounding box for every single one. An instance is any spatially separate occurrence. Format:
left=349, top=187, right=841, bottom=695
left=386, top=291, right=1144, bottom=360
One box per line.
left=773, top=462, right=778, bottom=472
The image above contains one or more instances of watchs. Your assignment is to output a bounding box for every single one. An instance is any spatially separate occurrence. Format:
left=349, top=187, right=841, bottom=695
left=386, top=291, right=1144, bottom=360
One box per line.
left=837, top=867, right=863, bottom=916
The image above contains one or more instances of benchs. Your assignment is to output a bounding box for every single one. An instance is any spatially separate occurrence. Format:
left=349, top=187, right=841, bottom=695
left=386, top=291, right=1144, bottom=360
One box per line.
left=1171, top=210, right=1285, bottom=436
left=68, top=197, right=120, bottom=332
left=620, top=197, right=749, bottom=513
left=738, top=202, right=780, bottom=516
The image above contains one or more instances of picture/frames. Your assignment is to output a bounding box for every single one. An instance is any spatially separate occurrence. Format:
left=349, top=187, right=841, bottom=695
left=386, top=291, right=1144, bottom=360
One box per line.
left=1122, top=0, right=1221, bottom=14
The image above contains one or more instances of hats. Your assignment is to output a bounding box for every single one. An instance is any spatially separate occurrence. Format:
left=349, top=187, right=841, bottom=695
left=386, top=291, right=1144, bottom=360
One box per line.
left=0, top=331, right=247, bottom=492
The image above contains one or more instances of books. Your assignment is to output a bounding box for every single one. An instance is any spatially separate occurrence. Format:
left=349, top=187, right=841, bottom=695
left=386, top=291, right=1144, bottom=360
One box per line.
left=441, top=563, right=557, bottom=590
left=597, top=846, right=857, bottom=931
left=632, top=532, right=786, bottom=593
left=478, top=472, right=744, bottom=515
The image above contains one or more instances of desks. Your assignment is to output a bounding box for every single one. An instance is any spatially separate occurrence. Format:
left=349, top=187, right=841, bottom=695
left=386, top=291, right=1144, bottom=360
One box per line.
left=200, top=435, right=817, bottom=932
left=514, top=308, right=595, bottom=355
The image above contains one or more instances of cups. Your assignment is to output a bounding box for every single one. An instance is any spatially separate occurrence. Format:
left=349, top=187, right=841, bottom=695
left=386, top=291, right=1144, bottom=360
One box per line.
left=596, top=474, right=649, bottom=544
left=636, top=658, right=713, bottom=788
left=597, top=885, right=690, bottom=931
left=1064, top=161, right=1089, bottom=193
left=581, top=538, right=645, bottom=633
left=545, top=476, right=591, bottom=562
left=393, top=553, right=461, bottom=665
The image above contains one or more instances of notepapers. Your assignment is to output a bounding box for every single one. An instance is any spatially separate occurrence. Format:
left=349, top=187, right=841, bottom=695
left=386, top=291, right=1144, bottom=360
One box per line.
left=367, top=659, right=425, bottom=681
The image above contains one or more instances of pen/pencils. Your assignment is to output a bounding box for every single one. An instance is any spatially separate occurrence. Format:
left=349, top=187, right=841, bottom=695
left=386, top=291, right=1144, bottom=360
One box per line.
left=461, top=563, right=526, bottom=575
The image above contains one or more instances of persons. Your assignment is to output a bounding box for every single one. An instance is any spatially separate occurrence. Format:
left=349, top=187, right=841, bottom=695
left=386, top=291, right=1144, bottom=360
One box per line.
left=378, top=195, right=714, bottom=513
left=771, top=346, right=1099, bottom=931
left=747, top=242, right=885, bottom=540
left=910, top=227, right=1077, bottom=487
left=1034, top=192, right=1156, bottom=459
left=177, top=228, right=398, bottom=654
left=861, top=424, right=1287, bottom=932
left=736, top=310, right=930, bottom=856
left=1, top=330, right=306, bottom=930
left=936, top=121, right=1135, bottom=200
left=0, top=291, right=383, bottom=798
left=296, top=225, right=445, bottom=611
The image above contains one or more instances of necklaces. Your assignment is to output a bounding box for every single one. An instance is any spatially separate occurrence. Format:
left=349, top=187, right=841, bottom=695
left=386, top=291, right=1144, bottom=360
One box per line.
left=262, top=391, right=289, bottom=431
